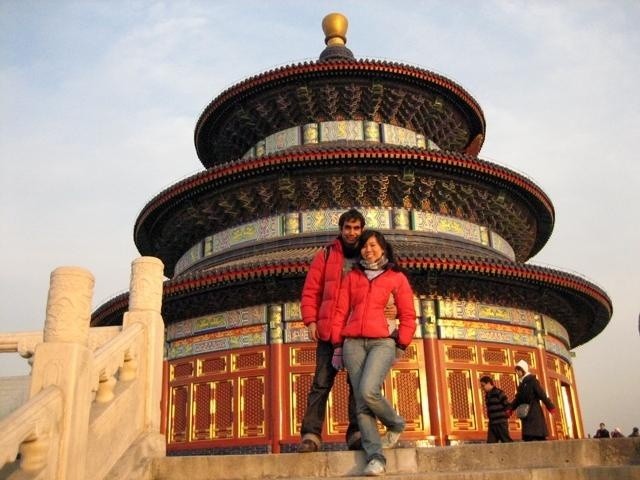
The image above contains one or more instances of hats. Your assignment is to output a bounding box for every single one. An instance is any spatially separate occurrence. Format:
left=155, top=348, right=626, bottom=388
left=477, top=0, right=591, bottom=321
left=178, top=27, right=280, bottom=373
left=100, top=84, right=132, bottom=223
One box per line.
left=516, top=359, right=529, bottom=374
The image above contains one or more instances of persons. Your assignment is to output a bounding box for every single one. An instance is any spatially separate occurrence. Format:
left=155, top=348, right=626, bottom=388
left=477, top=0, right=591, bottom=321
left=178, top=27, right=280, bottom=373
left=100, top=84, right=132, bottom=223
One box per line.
left=300, top=208, right=397, bottom=452
left=505, top=359, right=556, bottom=441
left=331, top=229, right=416, bottom=472
left=611, top=426, right=625, bottom=437
left=593, top=423, right=610, bottom=438
left=628, top=427, right=640, bottom=437
left=480, top=376, right=513, bottom=443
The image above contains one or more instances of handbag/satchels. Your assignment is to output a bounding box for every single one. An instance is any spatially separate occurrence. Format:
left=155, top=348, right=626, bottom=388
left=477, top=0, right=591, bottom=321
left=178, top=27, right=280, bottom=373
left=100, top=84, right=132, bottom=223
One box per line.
left=516, top=403, right=530, bottom=419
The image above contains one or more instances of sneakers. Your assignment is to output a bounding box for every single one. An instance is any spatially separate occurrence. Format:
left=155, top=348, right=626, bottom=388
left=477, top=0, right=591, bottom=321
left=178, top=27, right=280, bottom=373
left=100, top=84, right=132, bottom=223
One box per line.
left=297, top=432, right=322, bottom=453
left=348, top=431, right=367, bottom=450
left=380, top=429, right=401, bottom=449
left=362, top=458, right=386, bottom=476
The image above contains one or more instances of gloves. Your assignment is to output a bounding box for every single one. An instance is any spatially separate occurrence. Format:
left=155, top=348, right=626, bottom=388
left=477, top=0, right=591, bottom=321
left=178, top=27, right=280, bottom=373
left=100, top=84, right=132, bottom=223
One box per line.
left=503, top=407, right=514, bottom=417
left=549, top=406, right=558, bottom=416
left=392, top=345, right=404, bottom=368
left=331, top=346, right=346, bottom=370
